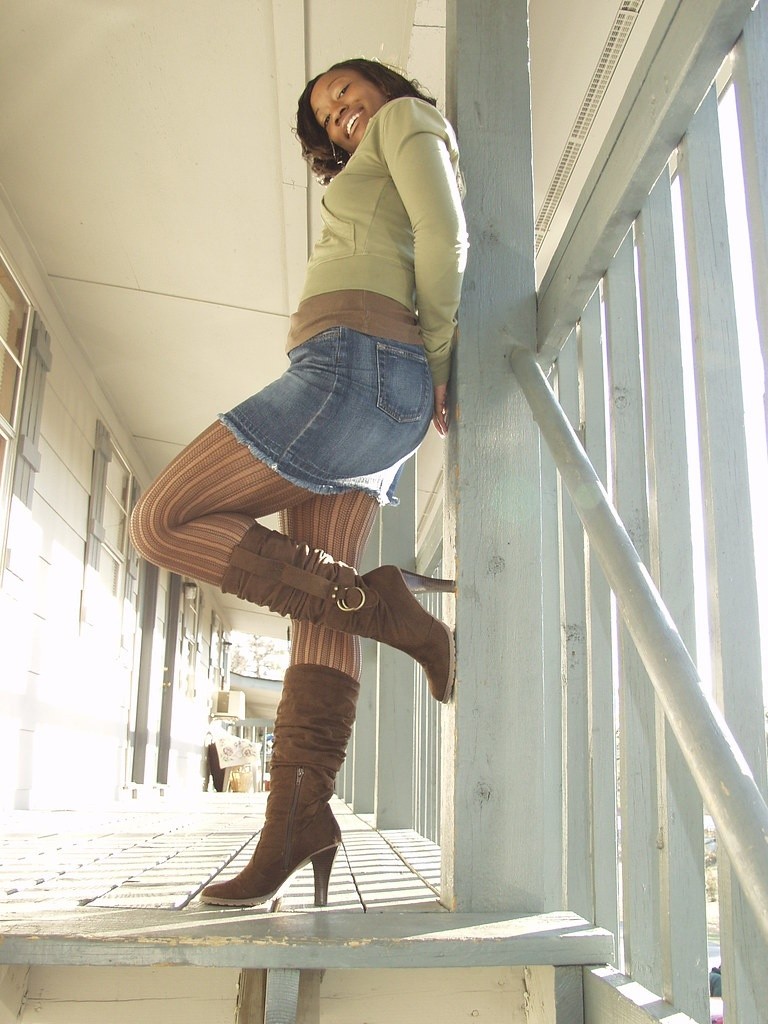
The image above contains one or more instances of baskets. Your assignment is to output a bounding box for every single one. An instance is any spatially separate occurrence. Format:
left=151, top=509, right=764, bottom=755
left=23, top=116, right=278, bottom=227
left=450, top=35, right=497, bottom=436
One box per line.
left=231, top=772, right=251, bottom=792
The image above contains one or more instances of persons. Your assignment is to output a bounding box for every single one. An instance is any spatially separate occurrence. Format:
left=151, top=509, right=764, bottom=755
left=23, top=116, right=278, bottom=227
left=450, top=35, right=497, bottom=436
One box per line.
left=129, top=58, right=472, bottom=906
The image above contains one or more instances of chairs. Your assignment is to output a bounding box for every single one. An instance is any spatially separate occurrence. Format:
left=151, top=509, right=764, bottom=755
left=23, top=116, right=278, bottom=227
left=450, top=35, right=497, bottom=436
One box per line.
left=222, top=718, right=275, bottom=792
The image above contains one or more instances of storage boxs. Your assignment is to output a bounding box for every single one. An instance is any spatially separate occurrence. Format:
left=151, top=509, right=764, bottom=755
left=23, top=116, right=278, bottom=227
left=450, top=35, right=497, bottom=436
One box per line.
left=217, top=690, right=246, bottom=718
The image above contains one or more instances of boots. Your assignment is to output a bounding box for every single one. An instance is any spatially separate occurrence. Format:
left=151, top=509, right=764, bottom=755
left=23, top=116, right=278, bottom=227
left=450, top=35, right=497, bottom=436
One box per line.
left=200, top=664, right=361, bottom=906
left=222, top=524, right=456, bottom=704
